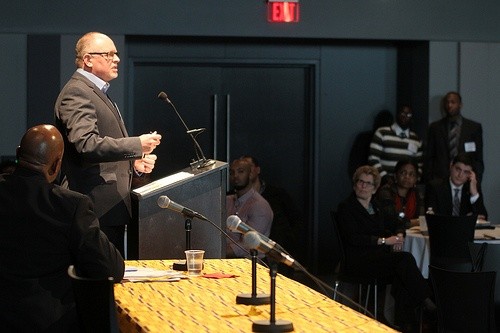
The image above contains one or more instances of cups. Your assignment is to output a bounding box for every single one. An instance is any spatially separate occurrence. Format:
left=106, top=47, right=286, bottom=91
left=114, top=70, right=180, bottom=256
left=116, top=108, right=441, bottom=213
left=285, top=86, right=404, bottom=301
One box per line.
left=419, top=216, right=428, bottom=232
left=185, top=250, right=205, bottom=276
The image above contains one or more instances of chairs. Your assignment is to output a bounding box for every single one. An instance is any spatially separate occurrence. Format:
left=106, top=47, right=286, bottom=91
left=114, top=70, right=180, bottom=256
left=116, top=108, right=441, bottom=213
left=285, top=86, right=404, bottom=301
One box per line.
left=326, top=208, right=391, bottom=320
left=428, top=265, right=497, bottom=333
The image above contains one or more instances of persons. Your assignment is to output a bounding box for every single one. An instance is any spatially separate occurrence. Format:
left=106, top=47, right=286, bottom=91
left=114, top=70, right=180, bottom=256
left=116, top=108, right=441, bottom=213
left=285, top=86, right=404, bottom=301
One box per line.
left=426, top=92, right=484, bottom=189
left=0, top=160, right=18, bottom=182
left=0, top=124, right=125, bottom=333
left=335, top=166, right=444, bottom=333
left=132, top=171, right=152, bottom=188
left=240, top=155, right=273, bottom=208
left=53, top=32, right=162, bottom=260
left=367, top=104, right=427, bottom=188
left=425, top=152, right=491, bottom=224
left=226, top=159, right=273, bottom=264
left=372, top=159, right=426, bottom=228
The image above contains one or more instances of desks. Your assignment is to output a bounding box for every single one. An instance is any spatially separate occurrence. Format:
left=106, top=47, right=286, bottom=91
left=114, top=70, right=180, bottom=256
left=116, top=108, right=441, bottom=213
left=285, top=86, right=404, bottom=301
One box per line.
left=114, top=259, right=401, bottom=333
left=404, top=222, right=500, bottom=333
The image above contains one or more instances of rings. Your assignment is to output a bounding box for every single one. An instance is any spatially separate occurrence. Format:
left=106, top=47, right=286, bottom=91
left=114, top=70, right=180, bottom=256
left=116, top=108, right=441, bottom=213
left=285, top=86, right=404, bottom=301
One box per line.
left=396, top=236, right=399, bottom=241
left=476, top=220, right=479, bottom=224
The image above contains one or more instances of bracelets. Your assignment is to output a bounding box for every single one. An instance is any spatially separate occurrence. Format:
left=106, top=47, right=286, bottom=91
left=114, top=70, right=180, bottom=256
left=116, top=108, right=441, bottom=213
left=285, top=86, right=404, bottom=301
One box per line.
left=382, top=238, right=386, bottom=245
left=417, top=219, right=420, bottom=225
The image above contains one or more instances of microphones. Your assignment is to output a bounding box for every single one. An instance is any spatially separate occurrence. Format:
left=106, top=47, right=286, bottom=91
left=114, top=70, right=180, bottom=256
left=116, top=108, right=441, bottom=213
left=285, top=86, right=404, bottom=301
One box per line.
left=158, top=91, right=216, bottom=169
left=157, top=195, right=208, bottom=221
left=226, top=215, right=290, bottom=256
left=244, top=231, right=301, bottom=270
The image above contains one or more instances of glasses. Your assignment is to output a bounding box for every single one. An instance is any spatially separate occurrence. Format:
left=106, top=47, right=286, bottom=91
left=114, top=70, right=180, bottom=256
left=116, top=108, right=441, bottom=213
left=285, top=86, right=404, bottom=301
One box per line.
left=400, top=112, right=413, bottom=118
left=359, top=179, right=375, bottom=186
left=88, top=51, right=119, bottom=59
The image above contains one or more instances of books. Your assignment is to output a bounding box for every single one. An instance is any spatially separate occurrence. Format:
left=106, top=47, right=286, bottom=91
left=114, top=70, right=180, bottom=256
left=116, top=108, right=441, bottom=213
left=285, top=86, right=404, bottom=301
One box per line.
left=473, top=224, right=500, bottom=245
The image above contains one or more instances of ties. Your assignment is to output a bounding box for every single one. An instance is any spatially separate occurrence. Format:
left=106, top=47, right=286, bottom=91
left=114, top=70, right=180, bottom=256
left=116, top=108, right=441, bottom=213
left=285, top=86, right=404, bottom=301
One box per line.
left=453, top=189, right=461, bottom=216
left=448, top=120, right=458, bottom=158
